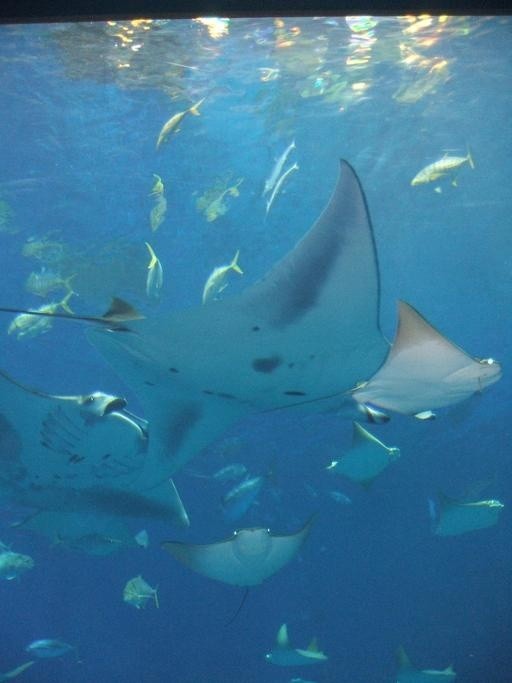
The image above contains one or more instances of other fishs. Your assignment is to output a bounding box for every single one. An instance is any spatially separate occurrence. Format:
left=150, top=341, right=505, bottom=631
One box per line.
left=0, top=139, right=503, bottom=619
